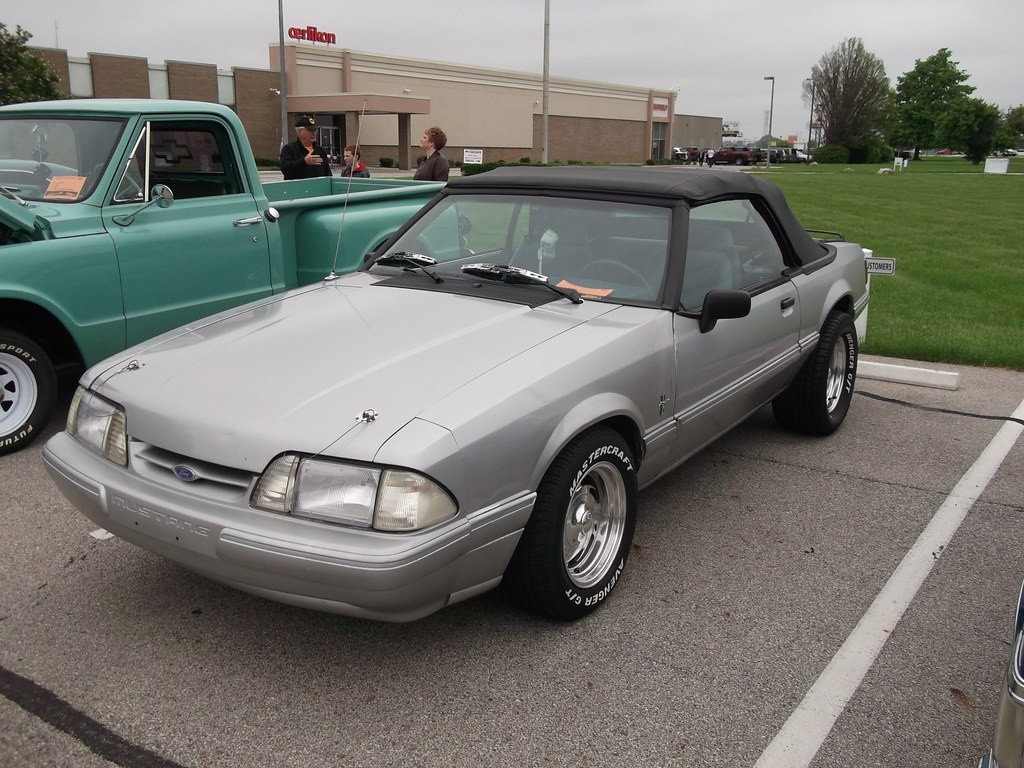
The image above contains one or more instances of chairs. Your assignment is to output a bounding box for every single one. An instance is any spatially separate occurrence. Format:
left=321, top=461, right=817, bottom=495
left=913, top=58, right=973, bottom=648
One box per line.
left=682, top=224, right=742, bottom=308
left=508, top=233, right=592, bottom=281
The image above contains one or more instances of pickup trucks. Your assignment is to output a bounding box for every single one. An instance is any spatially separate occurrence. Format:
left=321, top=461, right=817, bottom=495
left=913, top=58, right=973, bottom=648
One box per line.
left=0, top=97, right=472, bottom=460
left=706, top=146, right=757, bottom=167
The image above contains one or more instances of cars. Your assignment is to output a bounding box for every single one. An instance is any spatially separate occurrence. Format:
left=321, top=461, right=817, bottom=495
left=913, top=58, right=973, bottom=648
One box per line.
left=672, top=147, right=711, bottom=163
left=1003, top=148, right=1017, bottom=156
left=936, top=148, right=952, bottom=155
left=42, top=165, right=896, bottom=620
left=749, top=147, right=813, bottom=164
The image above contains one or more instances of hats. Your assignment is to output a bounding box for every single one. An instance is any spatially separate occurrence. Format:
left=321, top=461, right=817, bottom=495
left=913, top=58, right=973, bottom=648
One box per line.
left=295, top=114, right=320, bottom=131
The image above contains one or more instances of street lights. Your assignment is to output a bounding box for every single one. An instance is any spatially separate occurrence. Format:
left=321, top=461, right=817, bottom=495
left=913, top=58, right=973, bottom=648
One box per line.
left=806, top=78, right=815, bottom=166
left=764, top=76, right=775, bottom=169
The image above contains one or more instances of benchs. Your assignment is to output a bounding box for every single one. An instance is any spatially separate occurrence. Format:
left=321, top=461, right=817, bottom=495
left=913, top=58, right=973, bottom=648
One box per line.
left=150, top=177, right=233, bottom=199
left=607, top=237, right=748, bottom=296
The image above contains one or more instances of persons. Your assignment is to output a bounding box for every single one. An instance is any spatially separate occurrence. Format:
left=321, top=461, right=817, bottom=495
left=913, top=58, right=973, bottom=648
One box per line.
left=696, top=150, right=705, bottom=166
left=341, top=144, right=371, bottom=178
left=707, top=148, right=715, bottom=167
left=413, top=127, right=450, bottom=181
left=279, top=116, right=333, bottom=180
